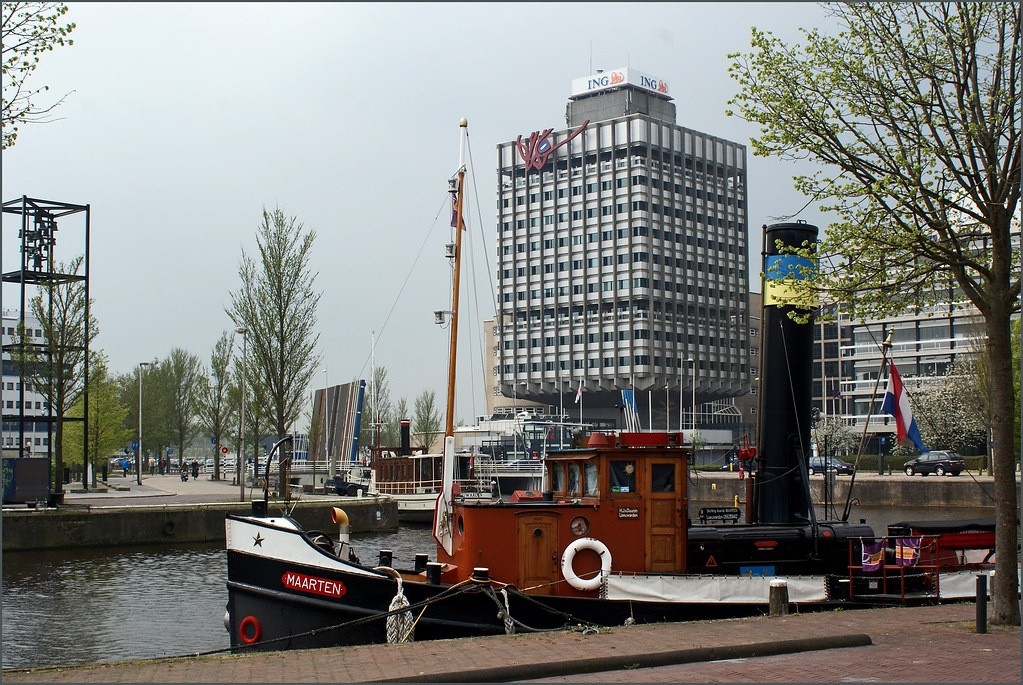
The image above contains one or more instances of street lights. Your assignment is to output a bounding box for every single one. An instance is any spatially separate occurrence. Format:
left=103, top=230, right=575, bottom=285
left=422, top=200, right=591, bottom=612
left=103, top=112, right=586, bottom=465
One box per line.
left=138, top=361, right=149, bottom=485
left=234, top=326, right=246, bottom=501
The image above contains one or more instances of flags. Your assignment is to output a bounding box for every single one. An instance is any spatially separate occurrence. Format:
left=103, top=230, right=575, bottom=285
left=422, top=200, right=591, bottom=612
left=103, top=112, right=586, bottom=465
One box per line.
left=879, top=356, right=929, bottom=454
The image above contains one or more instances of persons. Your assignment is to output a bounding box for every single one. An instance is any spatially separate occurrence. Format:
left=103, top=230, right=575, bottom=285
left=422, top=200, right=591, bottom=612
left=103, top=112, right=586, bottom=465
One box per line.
left=158, top=457, right=165, bottom=475
left=191, top=459, right=199, bottom=477
left=148, top=457, right=155, bottom=476
left=181, top=461, right=188, bottom=474
left=122, top=457, right=129, bottom=477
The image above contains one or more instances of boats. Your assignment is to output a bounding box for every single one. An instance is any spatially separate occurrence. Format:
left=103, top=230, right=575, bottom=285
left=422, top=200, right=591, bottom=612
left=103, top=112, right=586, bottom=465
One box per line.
left=223, top=118, right=1022, bottom=654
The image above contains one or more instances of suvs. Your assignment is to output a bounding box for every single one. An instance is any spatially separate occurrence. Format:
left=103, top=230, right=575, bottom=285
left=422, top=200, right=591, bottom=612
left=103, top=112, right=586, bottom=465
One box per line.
left=902, top=450, right=965, bottom=476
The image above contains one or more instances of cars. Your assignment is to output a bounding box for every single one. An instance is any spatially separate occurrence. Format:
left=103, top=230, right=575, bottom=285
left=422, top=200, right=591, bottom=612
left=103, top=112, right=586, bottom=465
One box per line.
left=111, top=457, right=265, bottom=468
left=808, top=455, right=855, bottom=475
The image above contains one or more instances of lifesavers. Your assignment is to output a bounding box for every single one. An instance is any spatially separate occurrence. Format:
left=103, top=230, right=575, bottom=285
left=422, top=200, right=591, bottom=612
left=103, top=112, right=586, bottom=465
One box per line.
left=561, top=538, right=612, bottom=589
left=239, top=616, right=261, bottom=644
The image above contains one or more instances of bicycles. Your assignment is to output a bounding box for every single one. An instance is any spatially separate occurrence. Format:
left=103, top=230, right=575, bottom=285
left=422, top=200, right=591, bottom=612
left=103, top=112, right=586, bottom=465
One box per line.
left=192, top=468, right=198, bottom=479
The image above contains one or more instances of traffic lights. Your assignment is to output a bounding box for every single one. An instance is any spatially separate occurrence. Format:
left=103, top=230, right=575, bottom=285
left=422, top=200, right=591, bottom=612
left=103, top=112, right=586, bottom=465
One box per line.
left=222, top=447, right=228, bottom=454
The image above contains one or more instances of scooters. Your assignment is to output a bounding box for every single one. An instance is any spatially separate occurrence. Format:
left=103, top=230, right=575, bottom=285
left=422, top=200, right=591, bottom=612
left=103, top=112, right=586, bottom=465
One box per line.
left=181, top=469, right=187, bottom=482
